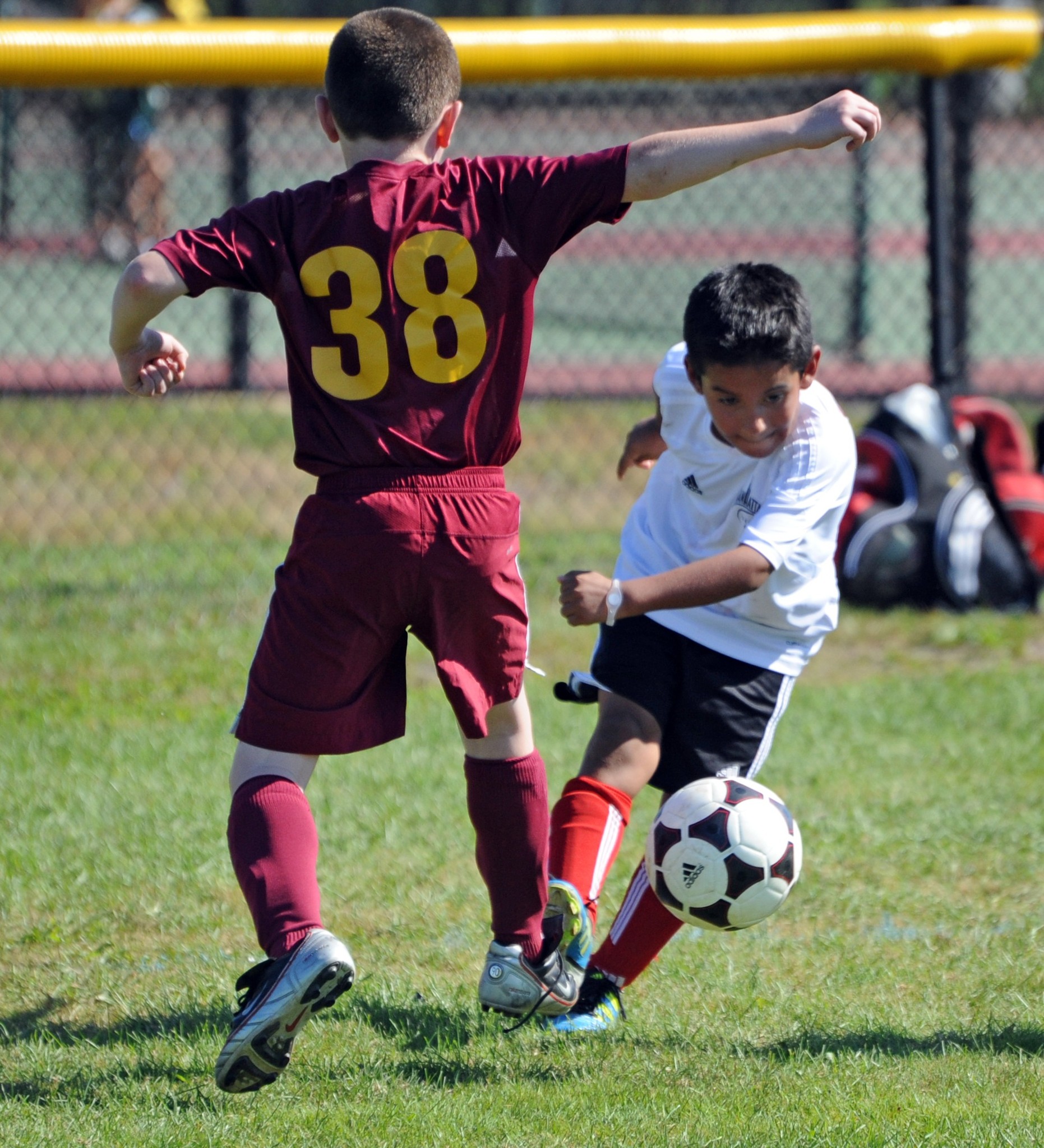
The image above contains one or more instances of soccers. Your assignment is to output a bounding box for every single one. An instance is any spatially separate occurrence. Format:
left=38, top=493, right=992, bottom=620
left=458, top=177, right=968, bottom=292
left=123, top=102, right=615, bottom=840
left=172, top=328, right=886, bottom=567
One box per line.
left=644, top=775, right=806, bottom=932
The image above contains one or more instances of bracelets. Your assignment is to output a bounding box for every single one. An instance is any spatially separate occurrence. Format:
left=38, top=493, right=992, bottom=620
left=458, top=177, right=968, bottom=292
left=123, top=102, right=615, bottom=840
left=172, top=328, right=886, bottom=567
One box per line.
left=605, top=578, right=625, bottom=626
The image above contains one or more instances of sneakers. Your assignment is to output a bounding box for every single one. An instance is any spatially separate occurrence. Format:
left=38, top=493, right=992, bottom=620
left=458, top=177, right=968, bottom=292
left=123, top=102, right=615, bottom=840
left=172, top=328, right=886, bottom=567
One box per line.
left=215, top=928, right=355, bottom=1093
left=541, top=880, right=594, bottom=972
left=540, top=968, right=627, bottom=1031
left=478, top=914, right=579, bottom=1033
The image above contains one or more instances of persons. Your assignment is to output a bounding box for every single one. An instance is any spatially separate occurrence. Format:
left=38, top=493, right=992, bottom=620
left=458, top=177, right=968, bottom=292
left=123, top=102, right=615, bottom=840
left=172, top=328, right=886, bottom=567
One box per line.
left=111, top=4, right=883, bottom=1098
left=532, top=261, right=859, bottom=1036
left=64, top=0, right=211, bottom=264
